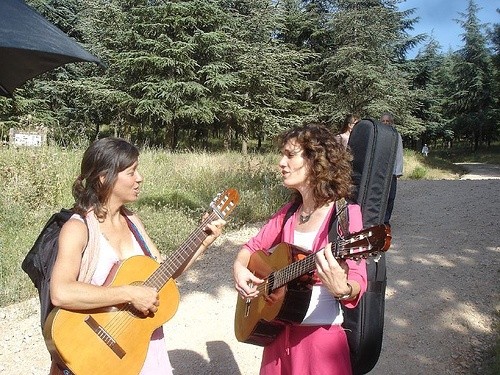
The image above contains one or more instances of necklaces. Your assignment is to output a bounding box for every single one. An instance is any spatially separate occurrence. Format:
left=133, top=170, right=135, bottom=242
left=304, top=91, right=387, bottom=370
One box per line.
left=299, top=203, right=319, bottom=223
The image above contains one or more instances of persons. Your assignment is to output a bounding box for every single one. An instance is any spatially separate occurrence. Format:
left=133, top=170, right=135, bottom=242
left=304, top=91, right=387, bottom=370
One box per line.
left=334, top=113, right=430, bottom=229
left=232, top=124, right=368, bottom=375
left=49, top=138, right=226, bottom=375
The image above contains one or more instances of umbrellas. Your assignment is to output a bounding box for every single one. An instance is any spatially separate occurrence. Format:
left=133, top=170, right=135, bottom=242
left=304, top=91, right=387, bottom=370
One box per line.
left=0, top=0, right=107, bottom=102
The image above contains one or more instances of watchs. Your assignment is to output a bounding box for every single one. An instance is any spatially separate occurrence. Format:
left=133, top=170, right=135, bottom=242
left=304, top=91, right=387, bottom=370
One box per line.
left=335, top=283, right=353, bottom=301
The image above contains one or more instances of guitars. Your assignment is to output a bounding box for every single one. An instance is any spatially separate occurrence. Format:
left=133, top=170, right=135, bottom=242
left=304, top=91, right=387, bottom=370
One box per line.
left=44, top=190, right=240, bottom=375
left=234, top=223, right=392, bottom=345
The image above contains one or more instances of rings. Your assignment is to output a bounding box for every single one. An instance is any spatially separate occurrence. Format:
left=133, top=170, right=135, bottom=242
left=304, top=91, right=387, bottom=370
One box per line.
left=239, top=289, right=244, bottom=295
left=149, top=305, right=154, bottom=309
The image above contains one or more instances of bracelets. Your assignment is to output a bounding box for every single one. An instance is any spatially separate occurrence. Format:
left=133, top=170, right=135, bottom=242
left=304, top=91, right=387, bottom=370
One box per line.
left=201, top=242, right=207, bottom=249
